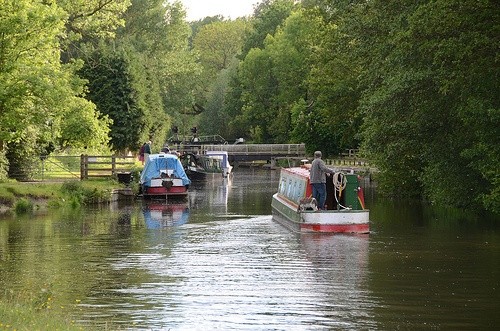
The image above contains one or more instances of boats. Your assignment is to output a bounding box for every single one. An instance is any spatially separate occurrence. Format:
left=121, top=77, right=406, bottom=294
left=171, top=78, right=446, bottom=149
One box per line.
left=138, top=154, right=192, bottom=201
left=271, top=159, right=370, bottom=234
left=140, top=201, right=190, bottom=230
left=163, top=144, right=233, bottom=180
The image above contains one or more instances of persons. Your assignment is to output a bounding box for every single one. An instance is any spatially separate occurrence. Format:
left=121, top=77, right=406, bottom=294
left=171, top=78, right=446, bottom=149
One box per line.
left=309, top=150, right=337, bottom=210
left=139, top=137, right=201, bottom=167
left=223, top=135, right=245, bottom=144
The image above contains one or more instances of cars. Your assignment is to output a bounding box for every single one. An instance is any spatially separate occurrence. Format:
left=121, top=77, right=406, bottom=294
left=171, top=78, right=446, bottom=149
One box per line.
left=168, top=135, right=244, bottom=145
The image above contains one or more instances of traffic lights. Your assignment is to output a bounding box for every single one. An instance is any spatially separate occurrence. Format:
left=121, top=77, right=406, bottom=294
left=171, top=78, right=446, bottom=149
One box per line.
left=191, top=127, right=197, bottom=133
left=172, top=127, right=178, bottom=133
